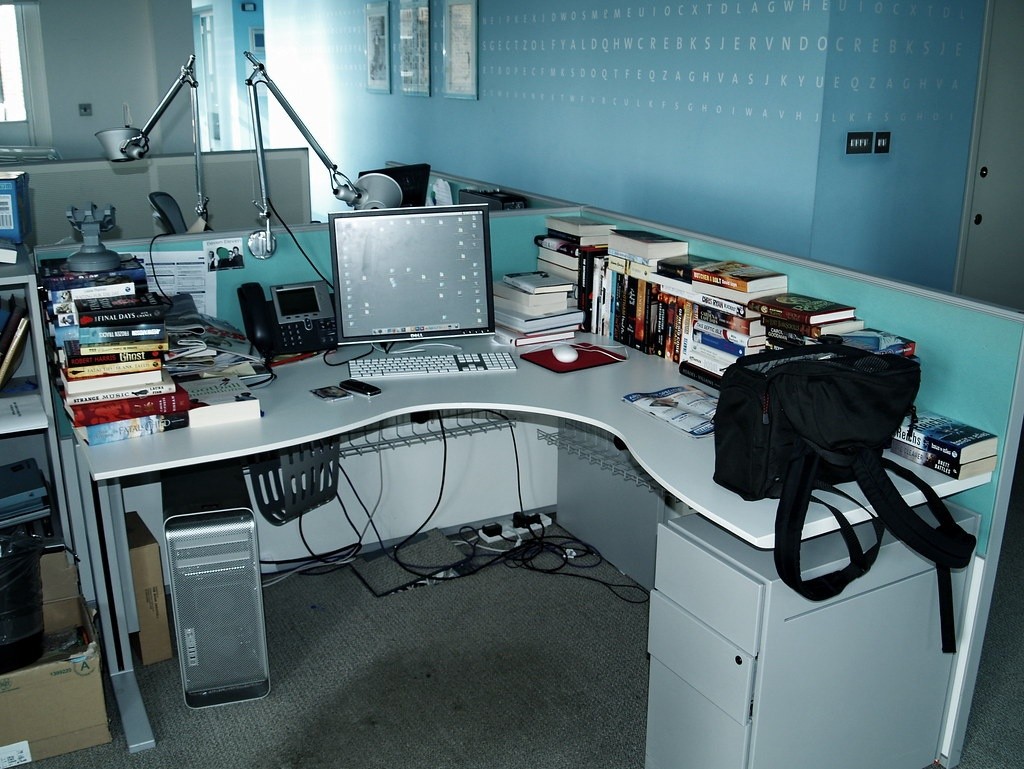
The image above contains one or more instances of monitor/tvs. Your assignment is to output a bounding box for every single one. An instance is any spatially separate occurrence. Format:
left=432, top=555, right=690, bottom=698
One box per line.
left=328, top=203, right=496, bottom=346
left=358, top=163, right=431, bottom=208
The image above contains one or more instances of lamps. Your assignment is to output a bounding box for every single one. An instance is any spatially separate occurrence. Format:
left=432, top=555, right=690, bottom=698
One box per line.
left=240, top=49, right=404, bottom=259
left=92, top=53, right=211, bottom=228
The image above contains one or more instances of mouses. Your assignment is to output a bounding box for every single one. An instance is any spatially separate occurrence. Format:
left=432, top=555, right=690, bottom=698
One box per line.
left=552, top=345, right=578, bottom=363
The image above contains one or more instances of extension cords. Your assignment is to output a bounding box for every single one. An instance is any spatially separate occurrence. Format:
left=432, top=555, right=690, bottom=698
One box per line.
left=479, top=512, right=552, bottom=543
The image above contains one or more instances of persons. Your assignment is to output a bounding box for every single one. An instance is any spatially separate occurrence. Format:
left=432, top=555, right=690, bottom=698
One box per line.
left=207, top=246, right=244, bottom=271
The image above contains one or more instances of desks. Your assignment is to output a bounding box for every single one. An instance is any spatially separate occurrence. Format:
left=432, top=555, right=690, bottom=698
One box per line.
left=77, top=332, right=992, bottom=769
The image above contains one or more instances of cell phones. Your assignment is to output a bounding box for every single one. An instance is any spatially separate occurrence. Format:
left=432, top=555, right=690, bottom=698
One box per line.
left=339, top=379, right=381, bottom=396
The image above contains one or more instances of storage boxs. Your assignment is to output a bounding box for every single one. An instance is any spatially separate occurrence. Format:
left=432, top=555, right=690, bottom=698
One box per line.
left=0, top=552, right=112, bottom=769
left=123, top=511, right=172, bottom=666
left=0, top=170, right=32, bottom=245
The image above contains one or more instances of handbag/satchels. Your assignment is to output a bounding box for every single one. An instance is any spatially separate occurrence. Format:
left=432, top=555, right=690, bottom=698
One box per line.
left=711, top=342, right=976, bottom=655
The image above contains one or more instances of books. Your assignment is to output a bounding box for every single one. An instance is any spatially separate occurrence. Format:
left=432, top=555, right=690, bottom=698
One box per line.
left=0, top=292, right=30, bottom=391
left=39, top=254, right=271, bottom=447
left=890, top=410, right=998, bottom=480
left=493, top=216, right=920, bottom=439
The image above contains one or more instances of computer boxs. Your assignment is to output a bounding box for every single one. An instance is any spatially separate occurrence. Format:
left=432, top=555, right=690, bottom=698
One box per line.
left=162, top=469, right=270, bottom=708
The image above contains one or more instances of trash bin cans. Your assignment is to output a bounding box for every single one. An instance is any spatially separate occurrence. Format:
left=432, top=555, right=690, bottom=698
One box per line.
left=0, top=535, right=44, bottom=674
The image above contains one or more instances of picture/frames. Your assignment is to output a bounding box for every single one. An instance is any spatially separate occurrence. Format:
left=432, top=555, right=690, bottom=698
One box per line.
left=247, top=26, right=264, bottom=55
left=441, top=0, right=480, bottom=101
left=364, top=0, right=393, bottom=95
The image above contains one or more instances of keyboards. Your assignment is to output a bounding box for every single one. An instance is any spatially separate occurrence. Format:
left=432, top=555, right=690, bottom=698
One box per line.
left=348, top=352, right=518, bottom=379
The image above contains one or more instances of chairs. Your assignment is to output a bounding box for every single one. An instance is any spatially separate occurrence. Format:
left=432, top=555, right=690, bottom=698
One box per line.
left=145, top=190, right=188, bottom=235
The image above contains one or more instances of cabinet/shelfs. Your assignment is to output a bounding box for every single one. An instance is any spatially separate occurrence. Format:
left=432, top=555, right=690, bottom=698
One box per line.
left=0, top=244, right=74, bottom=550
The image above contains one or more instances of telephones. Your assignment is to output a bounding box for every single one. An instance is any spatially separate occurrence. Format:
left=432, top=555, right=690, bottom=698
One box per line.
left=236, top=278, right=340, bottom=356
